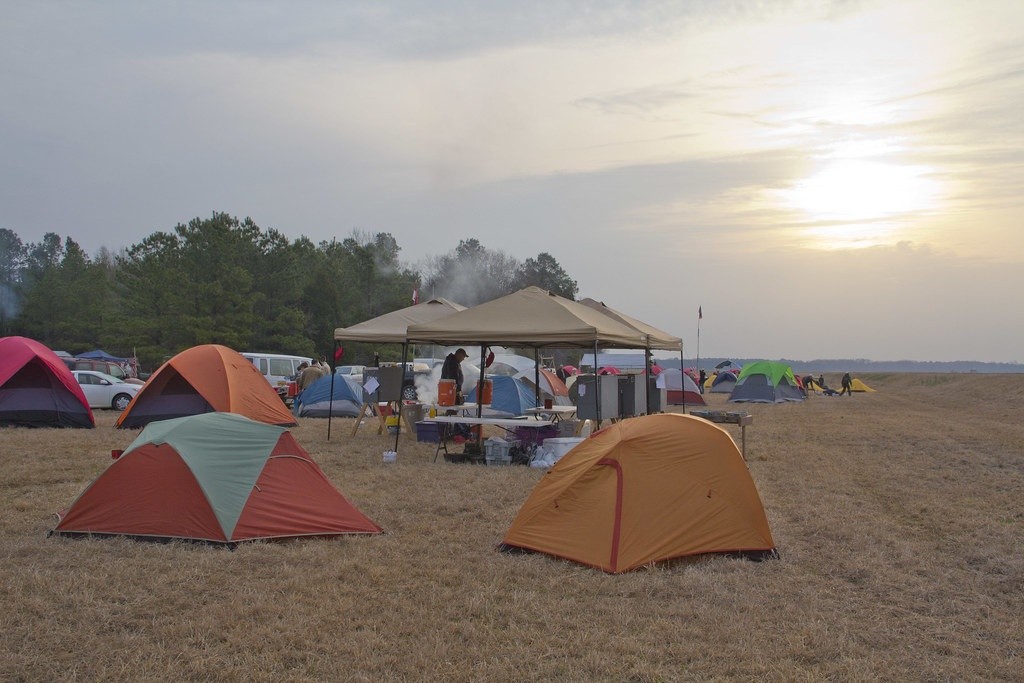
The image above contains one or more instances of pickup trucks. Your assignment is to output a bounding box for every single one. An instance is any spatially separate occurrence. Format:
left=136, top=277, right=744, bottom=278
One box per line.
left=400, top=371, right=432, bottom=401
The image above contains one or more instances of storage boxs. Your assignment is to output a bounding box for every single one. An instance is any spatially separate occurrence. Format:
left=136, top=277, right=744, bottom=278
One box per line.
left=416, top=421, right=442, bottom=443
left=464, top=439, right=477, bottom=452
left=452, top=453, right=465, bottom=462
left=483, top=440, right=520, bottom=456
left=486, top=455, right=514, bottom=466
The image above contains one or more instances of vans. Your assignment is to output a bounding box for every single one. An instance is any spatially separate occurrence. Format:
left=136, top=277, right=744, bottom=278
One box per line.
left=238, top=352, right=315, bottom=404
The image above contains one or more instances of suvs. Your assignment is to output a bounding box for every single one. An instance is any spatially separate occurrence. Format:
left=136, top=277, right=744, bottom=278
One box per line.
left=60, top=357, right=146, bottom=386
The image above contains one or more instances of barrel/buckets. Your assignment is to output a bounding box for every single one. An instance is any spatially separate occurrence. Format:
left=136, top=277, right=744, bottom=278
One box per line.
left=476, top=379, right=492, bottom=404
left=437, top=379, right=456, bottom=406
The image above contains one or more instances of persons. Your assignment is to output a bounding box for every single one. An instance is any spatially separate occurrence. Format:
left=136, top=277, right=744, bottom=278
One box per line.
left=440, top=348, right=470, bottom=404
left=801, top=374, right=814, bottom=392
left=698, top=369, right=706, bottom=394
left=557, top=365, right=570, bottom=383
left=838, top=371, right=853, bottom=397
left=818, top=374, right=824, bottom=388
left=571, top=368, right=577, bottom=376
left=296, top=355, right=330, bottom=392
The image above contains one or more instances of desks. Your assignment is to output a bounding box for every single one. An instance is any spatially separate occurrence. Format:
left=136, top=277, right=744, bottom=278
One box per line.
left=422, top=416, right=553, bottom=464
left=700, top=415, right=752, bottom=461
left=434, top=402, right=491, bottom=417
left=524, top=404, right=577, bottom=423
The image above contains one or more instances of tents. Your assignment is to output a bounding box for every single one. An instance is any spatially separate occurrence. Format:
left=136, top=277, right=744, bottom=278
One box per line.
left=46, top=410, right=386, bottom=546
left=295, top=374, right=372, bottom=416
left=497, top=413, right=782, bottom=576
left=332, top=284, right=873, bottom=464
left=0, top=335, right=136, bottom=430
left=111, top=344, right=299, bottom=427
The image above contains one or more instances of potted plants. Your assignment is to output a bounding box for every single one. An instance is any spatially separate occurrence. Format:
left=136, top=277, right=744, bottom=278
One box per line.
left=690, top=408, right=747, bottom=426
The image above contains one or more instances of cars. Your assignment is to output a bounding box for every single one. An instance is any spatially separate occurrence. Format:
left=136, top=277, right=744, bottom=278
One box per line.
left=335, top=365, right=366, bottom=387
left=71, top=370, right=143, bottom=411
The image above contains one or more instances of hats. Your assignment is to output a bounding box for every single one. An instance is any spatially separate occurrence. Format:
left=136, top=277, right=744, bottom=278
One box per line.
left=455, top=348, right=470, bottom=357
left=297, top=362, right=308, bottom=371
